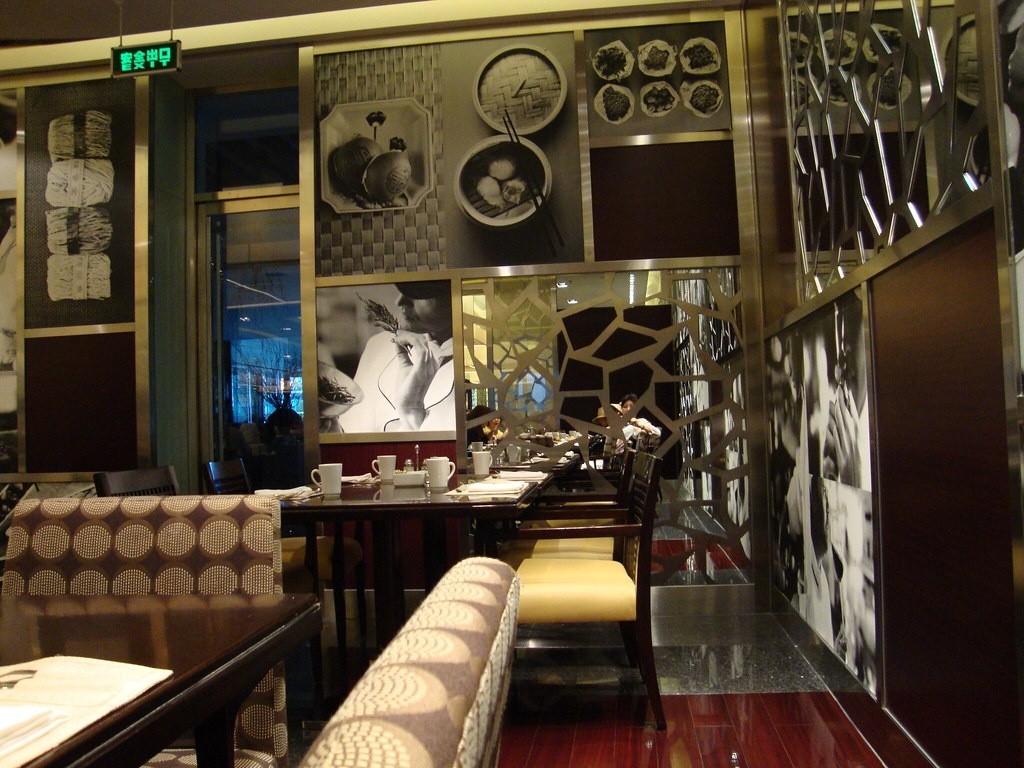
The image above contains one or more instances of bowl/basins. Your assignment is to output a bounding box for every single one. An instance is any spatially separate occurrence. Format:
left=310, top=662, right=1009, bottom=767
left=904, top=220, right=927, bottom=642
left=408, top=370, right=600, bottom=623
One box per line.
left=317, top=360, right=362, bottom=419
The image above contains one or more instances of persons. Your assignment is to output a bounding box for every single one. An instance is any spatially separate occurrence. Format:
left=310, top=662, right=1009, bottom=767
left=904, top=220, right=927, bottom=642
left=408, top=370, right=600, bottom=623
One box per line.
left=316, top=281, right=456, bottom=434
left=786, top=295, right=877, bottom=705
left=466, top=404, right=508, bottom=446
left=580, top=392, right=662, bottom=470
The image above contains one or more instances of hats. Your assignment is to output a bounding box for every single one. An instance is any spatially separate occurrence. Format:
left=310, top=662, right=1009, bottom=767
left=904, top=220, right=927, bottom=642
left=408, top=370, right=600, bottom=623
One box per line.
left=610, top=402, right=623, bottom=414
left=592, top=406, right=607, bottom=423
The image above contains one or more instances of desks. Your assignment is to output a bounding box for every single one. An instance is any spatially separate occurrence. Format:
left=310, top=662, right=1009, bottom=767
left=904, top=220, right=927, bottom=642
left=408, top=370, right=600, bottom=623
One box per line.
left=0, top=592, right=322, bottom=768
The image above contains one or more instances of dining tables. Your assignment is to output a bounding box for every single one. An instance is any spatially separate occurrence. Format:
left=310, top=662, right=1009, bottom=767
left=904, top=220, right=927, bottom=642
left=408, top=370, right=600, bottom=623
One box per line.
left=485, top=432, right=602, bottom=474
left=279, top=472, right=554, bottom=701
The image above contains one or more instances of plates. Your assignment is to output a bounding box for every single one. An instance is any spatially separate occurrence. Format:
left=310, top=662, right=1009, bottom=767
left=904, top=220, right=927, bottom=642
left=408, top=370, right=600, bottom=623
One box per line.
left=317, top=98, right=433, bottom=212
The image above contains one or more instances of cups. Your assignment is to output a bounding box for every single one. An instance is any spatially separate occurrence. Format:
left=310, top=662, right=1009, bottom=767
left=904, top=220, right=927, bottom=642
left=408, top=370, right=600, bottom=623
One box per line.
left=473, top=451, right=493, bottom=475
left=471, top=443, right=488, bottom=451
left=373, top=455, right=395, bottom=483
left=507, top=447, right=520, bottom=461
left=311, top=464, right=343, bottom=495
left=427, top=457, right=455, bottom=488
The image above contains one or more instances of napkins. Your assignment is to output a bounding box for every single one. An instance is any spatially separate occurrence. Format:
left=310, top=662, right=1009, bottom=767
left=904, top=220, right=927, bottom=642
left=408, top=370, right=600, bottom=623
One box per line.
left=499, top=471, right=543, bottom=479
left=470, top=483, right=523, bottom=492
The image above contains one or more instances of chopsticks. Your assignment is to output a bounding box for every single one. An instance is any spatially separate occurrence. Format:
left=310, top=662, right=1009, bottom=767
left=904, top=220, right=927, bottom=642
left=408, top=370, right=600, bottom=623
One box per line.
left=502, top=109, right=567, bottom=248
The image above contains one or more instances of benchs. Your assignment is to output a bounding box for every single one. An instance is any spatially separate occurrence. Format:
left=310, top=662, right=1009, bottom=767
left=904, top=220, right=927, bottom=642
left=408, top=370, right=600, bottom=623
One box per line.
left=3, top=494, right=290, bottom=768
left=296, top=556, right=520, bottom=768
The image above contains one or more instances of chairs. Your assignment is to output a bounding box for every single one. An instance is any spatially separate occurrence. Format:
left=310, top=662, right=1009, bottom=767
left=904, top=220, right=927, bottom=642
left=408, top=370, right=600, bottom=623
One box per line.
left=204, top=457, right=368, bottom=670
left=472, top=445, right=670, bottom=730
left=93, top=465, right=180, bottom=497
